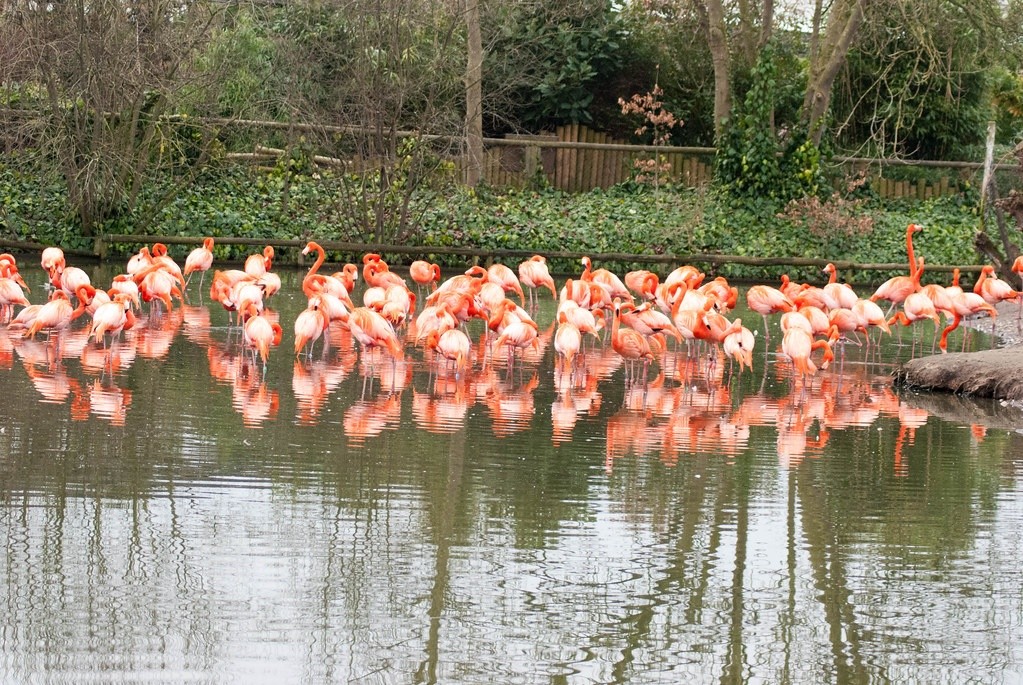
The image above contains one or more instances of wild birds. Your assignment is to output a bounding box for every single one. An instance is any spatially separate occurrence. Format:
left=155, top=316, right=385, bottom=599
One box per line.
left=0, top=330, right=987, bottom=480
left=0, top=223, right=1021, bottom=382
